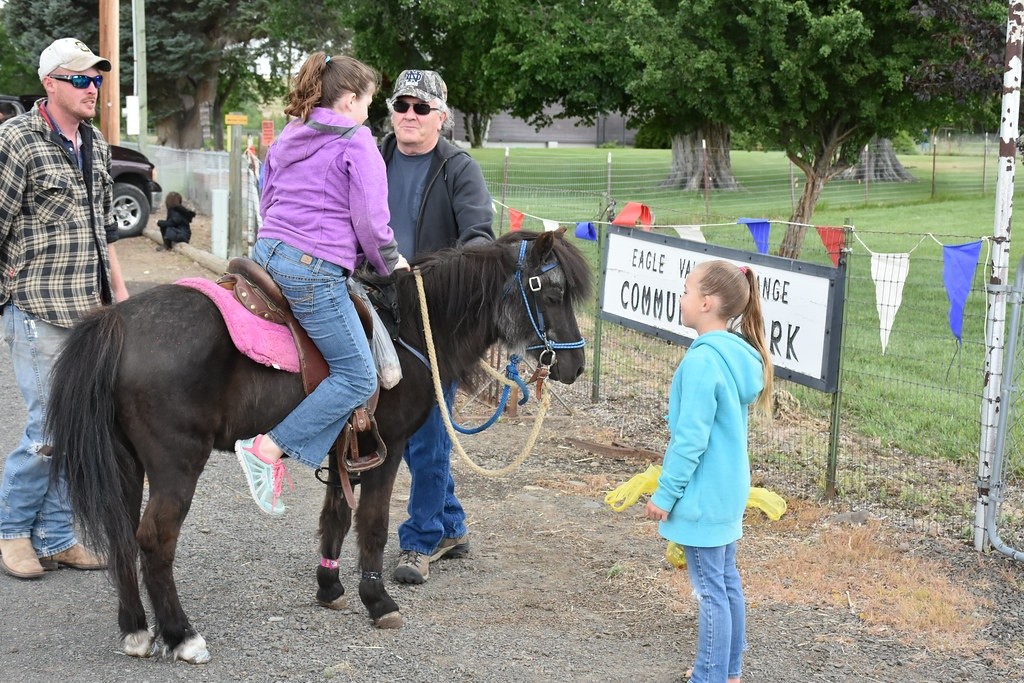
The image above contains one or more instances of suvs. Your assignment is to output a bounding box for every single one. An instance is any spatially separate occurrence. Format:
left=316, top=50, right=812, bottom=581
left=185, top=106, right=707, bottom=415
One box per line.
left=0, top=91, right=162, bottom=239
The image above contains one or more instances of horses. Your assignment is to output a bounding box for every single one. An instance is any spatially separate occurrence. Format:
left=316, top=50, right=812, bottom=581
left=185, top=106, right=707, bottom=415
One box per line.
left=39, top=225, right=594, bottom=663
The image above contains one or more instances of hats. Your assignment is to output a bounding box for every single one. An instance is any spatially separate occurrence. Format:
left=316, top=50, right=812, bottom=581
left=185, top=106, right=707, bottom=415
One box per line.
left=37, top=38, right=112, bottom=83
left=391, top=70, right=448, bottom=102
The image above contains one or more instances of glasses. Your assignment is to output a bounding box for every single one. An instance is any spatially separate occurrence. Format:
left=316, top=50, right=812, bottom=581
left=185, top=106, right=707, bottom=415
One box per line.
left=393, top=101, right=439, bottom=115
left=47, top=73, right=103, bottom=89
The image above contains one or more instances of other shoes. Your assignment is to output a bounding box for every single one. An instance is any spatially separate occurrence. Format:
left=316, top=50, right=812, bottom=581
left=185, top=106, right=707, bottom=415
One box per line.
left=680, top=667, right=740, bottom=683
left=156, top=244, right=172, bottom=252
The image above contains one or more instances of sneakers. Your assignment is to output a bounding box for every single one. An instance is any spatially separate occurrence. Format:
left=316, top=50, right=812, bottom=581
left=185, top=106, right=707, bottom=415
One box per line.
left=40, top=541, right=109, bottom=571
left=428, top=533, right=469, bottom=563
left=394, top=550, right=428, bottom=584
left=235, top=433, right=294, bottom=516
left=0, top=537, right=46, bottom=577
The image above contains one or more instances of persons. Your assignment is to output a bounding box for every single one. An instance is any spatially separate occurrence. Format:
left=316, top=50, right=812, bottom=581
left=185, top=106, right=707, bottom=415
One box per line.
left=0, top=38, right=129, bottom=578
left=156, top=192, right=195, bottom=250
left=377, top=70, right=496, bottom=583
left=235, top=51, right=410, bottom=516
left=644, top=259, right=773, bottom=683
